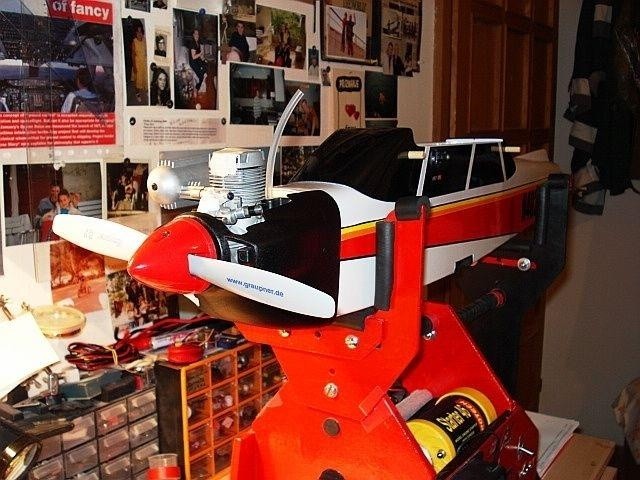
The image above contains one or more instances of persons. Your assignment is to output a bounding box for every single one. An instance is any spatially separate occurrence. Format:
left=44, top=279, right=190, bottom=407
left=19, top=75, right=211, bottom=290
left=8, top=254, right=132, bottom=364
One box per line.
left=123, top=275, right=171, bottom=325
left=42, top=191, right=82, bottom=227
left=61, top=70, right=100, bottom=116
left=189, top=29, right=207, bottom=99
left=308, top=52, right=318, bottom=75
left=374, top=92, right=390, bottom=117
left=231, top=22, right=249, bottom=60
left=253, top=90, right=260, bottom=123
left=346, top=11, right=356, bottom=57
left=383, top=42, right=393, bottom=74
left=322, top=68, right=331, bottom=85
left=113, top=171, right=143, bottom=211
left=130, top=24, right=147, bottom=102
left=342, top=13, right=346, bottom=50
left=281, top=21, right=291, bottom=68
left=149, top=69, right=169, bottom=105
left=154, top=36, right=165, bottom=56
left=301, top=101, right=318, bottom=136
left=35, top=183, right=59, bottom=223
left=394, top=43, right=407, bottom=75
